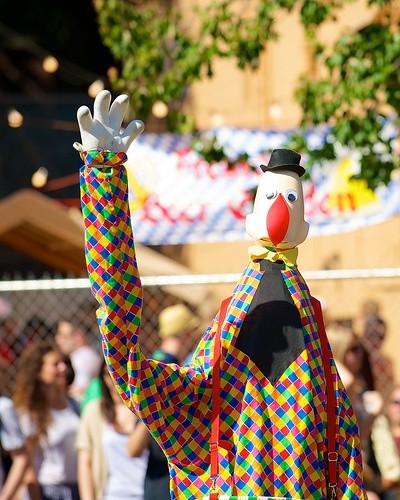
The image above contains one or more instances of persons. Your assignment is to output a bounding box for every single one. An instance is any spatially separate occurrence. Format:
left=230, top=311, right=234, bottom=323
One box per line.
left=1, top=394, right=31, bottom=499
left=149, top=301, right=201, bottom=368
left=125, top=419, right=171, bottom=500
left=11, top=340, right=84, bottom=500
left=325, top=298, right=399, bottom=499
left=76, top=355, right=149, bottom=499
left=55, top=314, right=102, bottom=403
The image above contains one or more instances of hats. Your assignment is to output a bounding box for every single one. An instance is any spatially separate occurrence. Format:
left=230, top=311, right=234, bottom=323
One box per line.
left=157, top=303, right=199, bottom=339
left=260, top=147, right=305, bottom=177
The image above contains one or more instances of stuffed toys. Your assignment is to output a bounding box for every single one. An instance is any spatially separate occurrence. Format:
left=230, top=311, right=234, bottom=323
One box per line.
left=70, top=88, right=369, bottom=500
